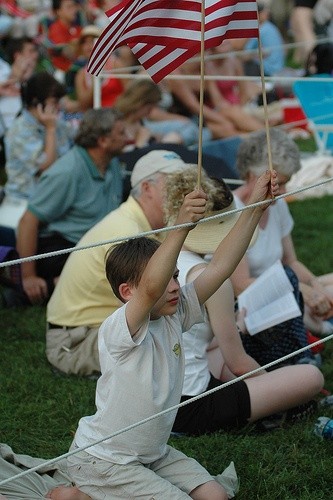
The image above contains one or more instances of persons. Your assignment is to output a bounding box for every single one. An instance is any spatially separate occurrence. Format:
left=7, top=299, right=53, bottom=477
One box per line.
left=0, top=0, right=333, bottom=309
left=200, top=126, right=333, bottom=339
left=139, top=171, right=326, bottom=438
left=45, top=147, right=191, bottom=378
left=40, top=169, right=279, bottom=500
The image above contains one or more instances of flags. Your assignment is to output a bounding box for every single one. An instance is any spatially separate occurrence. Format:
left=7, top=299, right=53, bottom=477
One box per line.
left=85, top=0, right=207, bottom=77
left=104, top=1, right=263, bottom=87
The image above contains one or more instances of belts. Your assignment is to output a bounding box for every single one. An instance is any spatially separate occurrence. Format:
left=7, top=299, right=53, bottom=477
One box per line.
left=48, top=322, right=78, bottom=331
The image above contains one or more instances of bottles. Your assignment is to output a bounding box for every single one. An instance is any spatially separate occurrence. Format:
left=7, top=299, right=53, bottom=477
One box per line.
left=313, top=416, right=333, bottom=440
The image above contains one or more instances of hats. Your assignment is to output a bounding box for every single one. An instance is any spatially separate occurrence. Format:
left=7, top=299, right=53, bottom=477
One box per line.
left=78, top=25, right=102, bottom=45
left=131, top=149, right=205, bottom=191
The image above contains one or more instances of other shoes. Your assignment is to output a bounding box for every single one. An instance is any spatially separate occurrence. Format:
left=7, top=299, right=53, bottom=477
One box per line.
left=272, top=399, right=316, bottom=419
